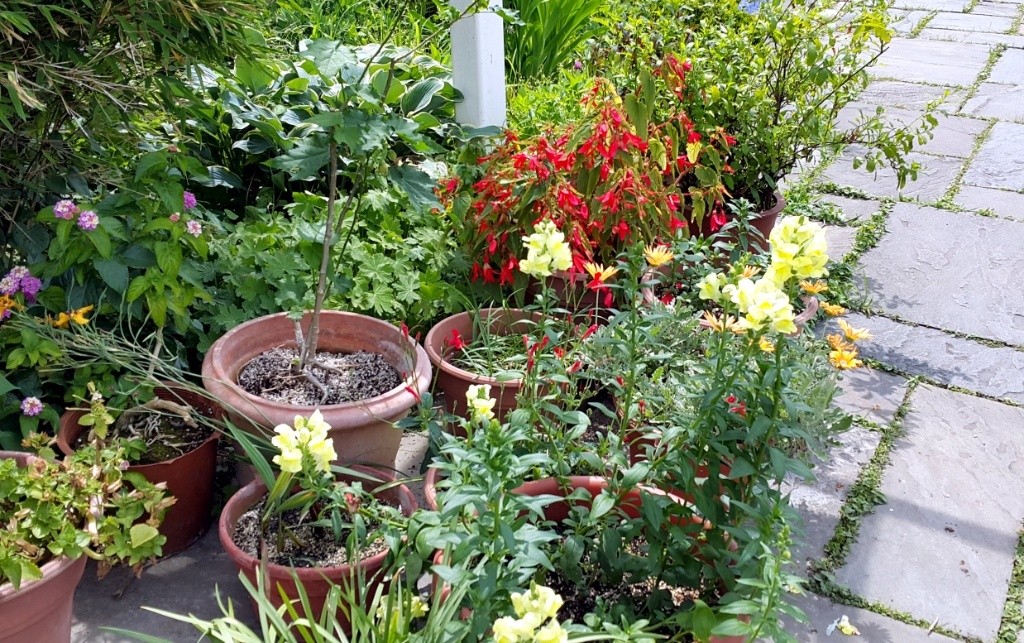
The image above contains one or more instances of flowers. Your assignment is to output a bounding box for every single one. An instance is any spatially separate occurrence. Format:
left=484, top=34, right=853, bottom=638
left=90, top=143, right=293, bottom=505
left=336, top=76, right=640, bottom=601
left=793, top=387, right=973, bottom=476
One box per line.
left=0, top=52, right=875, bottom=643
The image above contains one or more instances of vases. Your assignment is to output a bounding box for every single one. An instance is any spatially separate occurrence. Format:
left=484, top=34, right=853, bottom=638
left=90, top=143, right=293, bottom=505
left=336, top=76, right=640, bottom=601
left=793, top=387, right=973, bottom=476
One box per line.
left=614, top=393, right=761, bottom=527
left=431, top=475, right=748, bottom=643
left=202, top=308, right=431, bottom=481
left=0, top=450, right=99, bottom=643
left=641, top=262, right=819, bottom=361
left=220, top=460, right=419, bottom=643
left=425, top=307, right=593, bottom=461
left=425, top=462, right=443, bottom=513
left=57, top=383, right=225, bottom=556
left=525, top=257, right=629, bottom=318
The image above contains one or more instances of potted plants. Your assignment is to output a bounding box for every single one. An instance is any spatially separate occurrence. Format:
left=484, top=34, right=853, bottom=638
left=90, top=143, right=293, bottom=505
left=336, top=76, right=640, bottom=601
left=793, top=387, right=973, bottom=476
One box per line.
left=180, top=23, right=432, bottom=480
left=666, top=0, right=948, bottom=275
